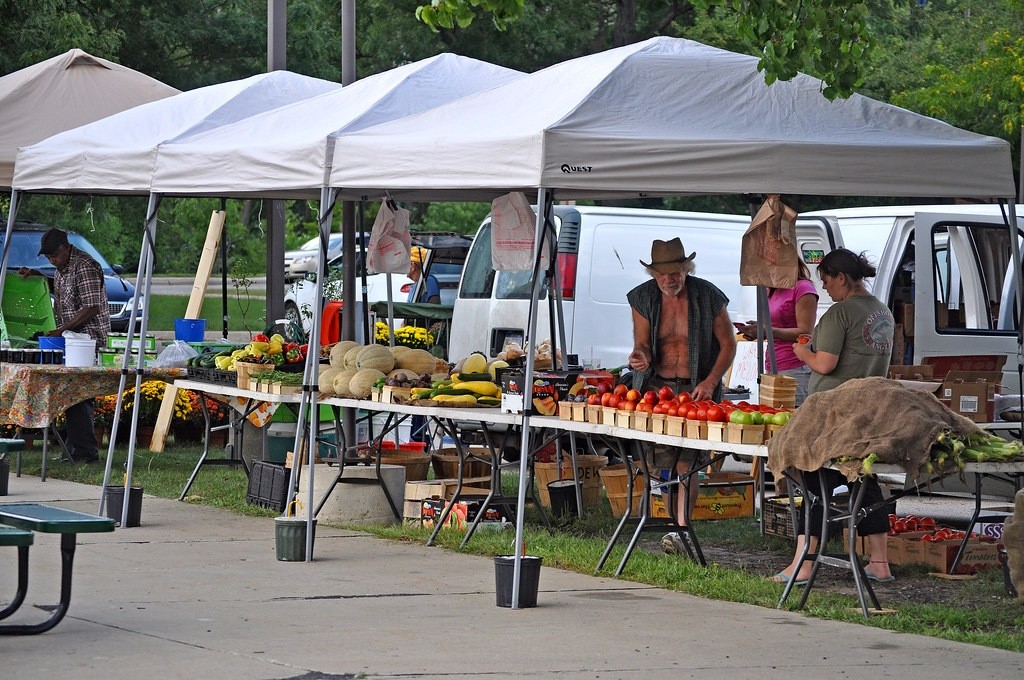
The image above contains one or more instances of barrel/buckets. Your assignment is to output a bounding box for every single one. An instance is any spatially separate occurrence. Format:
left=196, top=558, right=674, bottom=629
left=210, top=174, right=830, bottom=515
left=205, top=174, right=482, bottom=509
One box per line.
left=62, top=333, right=91, bottom=341
left=106, top=486, right=143, bottom=528
left=39, top=337, right=65, bottom=363
left=375, top=442, right=426, bottom=453
left=275, top=517, right=317, bottom=561
left=65, top=340, right=96, bottom=368
left=0, top=459, right=10, bottom=496
left=547, top=479, right=584, bottom=518
left=174, top=319, right=206, bottom=342
left=494, top=555, right=542, bottom=608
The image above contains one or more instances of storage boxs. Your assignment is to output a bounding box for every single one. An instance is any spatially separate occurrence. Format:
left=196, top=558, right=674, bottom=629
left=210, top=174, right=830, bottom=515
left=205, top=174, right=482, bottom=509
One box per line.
left=272, top=385, right=302, bottom=395
left=650, top=471, right=756, bottom=520
left=97, top=334, right=158, bottom=366
left=0, top=270, right=57, bottom=349
left=262, top=383, right=272, bottom=394
left=187, top=366, right=237, bottom=387
left=843, top=506, right=1014, bottom=574
left=246, top=403, right=345, bottom=513
left=404, top=477, right=513, bottom=532
left=249, top=382, right=262, bottom=391
left=763, top=493, right=843, bottom=540
left=495, top=356, right=615, bottom=415
left=889, top=302, right=999, bottom=423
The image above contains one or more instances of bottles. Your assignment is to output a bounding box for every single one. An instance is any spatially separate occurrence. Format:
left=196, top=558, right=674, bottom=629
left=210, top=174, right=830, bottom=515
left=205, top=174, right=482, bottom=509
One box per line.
left=131, top=333, right=155, bottom=350
left=129, top=349, right=138, bottom=367
left=98, top=348, right=125, bottom=367
left=106, top=332, right=128, bottom=349
left=144, top=350, right=157, bottom=368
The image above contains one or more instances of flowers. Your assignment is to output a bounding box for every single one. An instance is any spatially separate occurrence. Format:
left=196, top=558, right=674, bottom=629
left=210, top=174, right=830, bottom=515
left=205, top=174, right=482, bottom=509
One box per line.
left=0, top=379, right=230, bottom=436
left=375, top=321, right=446, bottom=350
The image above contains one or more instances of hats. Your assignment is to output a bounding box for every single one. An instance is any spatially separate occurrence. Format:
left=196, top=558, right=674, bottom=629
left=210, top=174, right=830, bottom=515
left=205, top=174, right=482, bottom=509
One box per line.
left=38, top=228, right=68, bottom=256
left=410, top=246, right=427, bottom=263
left=639, top=237, right=696, bottom=274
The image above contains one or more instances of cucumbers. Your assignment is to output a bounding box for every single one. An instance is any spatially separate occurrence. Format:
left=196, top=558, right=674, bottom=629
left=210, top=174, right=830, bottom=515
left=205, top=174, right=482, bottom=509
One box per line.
left=187, top=344, right=240, bottom=368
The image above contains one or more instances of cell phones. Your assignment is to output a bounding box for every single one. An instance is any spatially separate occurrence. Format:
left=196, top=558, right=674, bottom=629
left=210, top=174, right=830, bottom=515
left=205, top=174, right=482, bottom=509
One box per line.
left=732, top=322, right=747, bottom=338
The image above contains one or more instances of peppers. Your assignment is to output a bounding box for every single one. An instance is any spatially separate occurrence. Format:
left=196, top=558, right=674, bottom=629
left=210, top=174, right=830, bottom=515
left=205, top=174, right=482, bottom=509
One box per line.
left=215, top=334, right=308, bottom=371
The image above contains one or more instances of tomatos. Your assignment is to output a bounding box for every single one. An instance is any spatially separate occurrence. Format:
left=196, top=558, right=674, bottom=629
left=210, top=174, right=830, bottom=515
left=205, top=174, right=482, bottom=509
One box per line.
left=645, top=384, right=794, bottom=425
left=887, top=513, right=976, bottom=542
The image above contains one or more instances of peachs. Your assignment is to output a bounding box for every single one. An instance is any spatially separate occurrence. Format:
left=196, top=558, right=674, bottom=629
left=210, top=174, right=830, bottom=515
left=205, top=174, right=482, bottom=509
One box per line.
left=589, top=380, right=644, bottom=411
left=533, top=396, right=556, bottom=415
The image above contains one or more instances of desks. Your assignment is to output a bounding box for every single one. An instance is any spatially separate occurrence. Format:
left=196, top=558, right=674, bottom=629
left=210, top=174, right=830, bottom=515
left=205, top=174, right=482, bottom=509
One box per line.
left=162, top=341, right=250, bottom=354
left=515, top=415, right=770, bottom=576
left=174, top=379, right=403, bottom=520
left=0, top=361, right=188, bottom=482
left=977, top=420, right=1024, bottom=442
left=797, top=460, right=1024, bottom=620
left=335, top=397, right=556, bottom=549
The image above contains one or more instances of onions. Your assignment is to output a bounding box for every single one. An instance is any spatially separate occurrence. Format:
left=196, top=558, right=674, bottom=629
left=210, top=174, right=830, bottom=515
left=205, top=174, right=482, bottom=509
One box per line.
left=507, top=338, right=561, bottom=360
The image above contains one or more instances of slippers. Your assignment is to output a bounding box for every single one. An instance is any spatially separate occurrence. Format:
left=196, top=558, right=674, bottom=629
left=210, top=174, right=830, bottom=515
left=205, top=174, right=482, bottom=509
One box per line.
left=865, top=568, right=896, bottom=583
left=766, top=571, right=809, bottom=586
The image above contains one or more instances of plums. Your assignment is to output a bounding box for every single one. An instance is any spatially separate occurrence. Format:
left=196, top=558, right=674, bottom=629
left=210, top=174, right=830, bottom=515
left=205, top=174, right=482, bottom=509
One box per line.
left=566, top=393, right=588, bottom=402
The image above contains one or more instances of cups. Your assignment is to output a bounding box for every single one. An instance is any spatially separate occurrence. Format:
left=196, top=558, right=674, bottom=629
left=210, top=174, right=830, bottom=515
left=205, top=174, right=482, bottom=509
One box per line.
left=567, top=354, right=578, bottom=365
left=583, top=359, right=601, bottom=371
left=798, top=334, right=812, bottom=344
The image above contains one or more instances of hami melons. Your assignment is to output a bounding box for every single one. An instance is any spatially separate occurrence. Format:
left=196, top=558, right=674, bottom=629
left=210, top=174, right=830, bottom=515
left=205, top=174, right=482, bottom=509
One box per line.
left=319, top=340, right=437, bottom=398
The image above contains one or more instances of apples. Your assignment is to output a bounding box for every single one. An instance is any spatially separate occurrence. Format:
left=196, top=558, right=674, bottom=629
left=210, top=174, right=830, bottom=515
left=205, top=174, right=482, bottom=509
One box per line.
left=798, top=335, right=812, bottom=343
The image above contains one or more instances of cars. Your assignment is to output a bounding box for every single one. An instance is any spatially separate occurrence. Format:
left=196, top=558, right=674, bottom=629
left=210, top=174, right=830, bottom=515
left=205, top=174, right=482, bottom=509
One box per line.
left=0, top=218, right=143, bottom=333
left=285, top=231, right=370, bottom=284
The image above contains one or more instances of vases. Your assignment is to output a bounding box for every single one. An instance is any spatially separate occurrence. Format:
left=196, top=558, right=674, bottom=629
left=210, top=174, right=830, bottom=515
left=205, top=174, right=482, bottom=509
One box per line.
left=48, top=433, right=67, bottom=446
left=104, top=427, right=111, bottom=439
left=209, top=428, right=226, bottom=447
left=94, top=426, right=104, bottom=446
left=137, top=426, right=155, bottom=446
left=21, top=434, right=34, bottom=448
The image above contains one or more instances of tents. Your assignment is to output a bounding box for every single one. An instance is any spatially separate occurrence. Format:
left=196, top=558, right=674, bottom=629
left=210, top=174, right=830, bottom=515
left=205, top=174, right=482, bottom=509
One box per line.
left=0, top=36, right=1024, bottom=610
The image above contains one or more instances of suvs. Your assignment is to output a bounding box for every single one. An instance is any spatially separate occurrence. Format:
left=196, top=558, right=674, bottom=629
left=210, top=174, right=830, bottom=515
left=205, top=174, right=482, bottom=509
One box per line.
left=283, top=228, right=474, bottom=349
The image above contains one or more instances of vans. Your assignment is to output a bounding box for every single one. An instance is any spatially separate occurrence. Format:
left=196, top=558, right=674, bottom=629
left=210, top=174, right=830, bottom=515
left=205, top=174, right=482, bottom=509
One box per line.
left=447, top=206, right=769, bottom=405
left=794, top=203, right=1023, bottom=395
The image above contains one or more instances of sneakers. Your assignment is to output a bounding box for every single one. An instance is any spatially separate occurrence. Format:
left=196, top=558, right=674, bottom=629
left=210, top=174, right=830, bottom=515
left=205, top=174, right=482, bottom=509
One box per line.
left=662, top=531, right=689, bottom=556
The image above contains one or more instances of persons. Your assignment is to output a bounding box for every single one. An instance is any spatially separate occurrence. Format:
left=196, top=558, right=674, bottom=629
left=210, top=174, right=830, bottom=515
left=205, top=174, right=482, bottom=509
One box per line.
left=736, top=254, right=819, bottom=495
left=772, top=250, right=895, bottom=583
left=15, top=228, right=111, bottom=464
left=626, top=237, right=738, bottom=555
left=401, top=246, right=441, bottom=329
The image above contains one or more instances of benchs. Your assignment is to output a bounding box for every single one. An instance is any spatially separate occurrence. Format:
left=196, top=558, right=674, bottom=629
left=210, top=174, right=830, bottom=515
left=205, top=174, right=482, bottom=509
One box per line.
left=0, top=438, right=25, bottom=459
left=0, top=503, right=116, bottom=636
